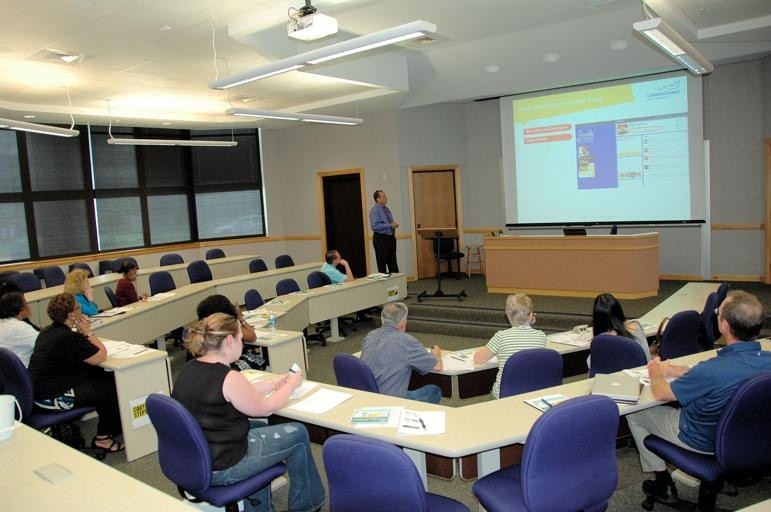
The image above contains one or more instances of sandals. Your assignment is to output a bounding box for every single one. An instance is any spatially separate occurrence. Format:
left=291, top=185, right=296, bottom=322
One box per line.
left=90, top=434, right=125, bottom=453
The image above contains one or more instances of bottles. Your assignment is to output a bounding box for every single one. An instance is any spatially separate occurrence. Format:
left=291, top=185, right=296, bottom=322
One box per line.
left=268, top=315, right=277, bottom=337
left=650, top=341, right=658, bottom=360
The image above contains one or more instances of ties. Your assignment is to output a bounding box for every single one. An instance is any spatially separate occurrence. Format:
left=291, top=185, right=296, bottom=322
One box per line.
left=383, top=206, right=391, bottom=223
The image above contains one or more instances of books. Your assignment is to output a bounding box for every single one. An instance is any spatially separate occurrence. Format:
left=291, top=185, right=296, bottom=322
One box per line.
left=524, top=393, right=570, bottom=413
left=240, top=368, right=270, bottom=382
left=347, top=404, right=404, bottom=428
left=585, top=373, right=645, bottom=405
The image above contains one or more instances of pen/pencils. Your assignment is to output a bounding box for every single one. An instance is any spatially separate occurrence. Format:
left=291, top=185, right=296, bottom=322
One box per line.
left=541, top=398, right=553, bottom=408
left=289, top=368, right=296, bottom=373
left=135, top=349, right=148, bottom=354
left=451, top=356, right=465, bottom=362
left=420, top=419, right=426, bottom=429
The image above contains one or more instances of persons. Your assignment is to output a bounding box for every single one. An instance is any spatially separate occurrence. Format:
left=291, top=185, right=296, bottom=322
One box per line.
left=184, top=293, right=266, bottom=371
left=359, top=300, right=444, bottom=406
left=0, top=281, right=42, bottom=332
left=473, top=292, right=549, bottom=401
left=586, top=293, right=652, bottom=369
left=171, top=310, right=327, bottom=511
left=64, top=267, right=99, bottom=316
left=114, top=260, right=147, bottom=306
left=369, top=189, right=402, bottom=274
left=320, top=249, right=374, bottom=323
left=0, top=292, right=42, bottom=369
left=28, top=292, right=126, bottom=452
left=626, top=288, right=771, bottom=503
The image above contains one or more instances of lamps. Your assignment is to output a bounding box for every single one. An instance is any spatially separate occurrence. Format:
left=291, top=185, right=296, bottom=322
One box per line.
left=101, top=96, right=240, bottom=149
left=630, top=1, right=717, bottom=78
left=0, top=53, right=81, bottom=140
left=221, top=58, right=366, bottom=129
left=207, top=11, right=442, bottom=92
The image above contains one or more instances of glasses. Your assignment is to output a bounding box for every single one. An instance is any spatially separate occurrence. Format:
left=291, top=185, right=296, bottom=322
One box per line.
left=713, top=308, right=720, bottom=316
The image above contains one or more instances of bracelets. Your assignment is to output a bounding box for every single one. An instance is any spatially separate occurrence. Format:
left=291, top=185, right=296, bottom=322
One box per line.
left=84, top=331, right=95, bottom=340
left=242, top=320, right=246, bottom=325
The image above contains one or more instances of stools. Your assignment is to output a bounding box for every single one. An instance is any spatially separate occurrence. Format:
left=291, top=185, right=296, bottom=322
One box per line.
left=464, top=243, right=486, bottom=279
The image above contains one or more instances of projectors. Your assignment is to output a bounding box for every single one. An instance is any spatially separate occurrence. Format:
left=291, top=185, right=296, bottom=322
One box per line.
left=287, top=13, right=339, bottom=40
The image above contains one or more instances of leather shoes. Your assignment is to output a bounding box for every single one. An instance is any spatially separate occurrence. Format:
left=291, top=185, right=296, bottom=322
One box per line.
left=641, top=478, right=679, bottom=504
left=359, top=317, right=375, bottom=323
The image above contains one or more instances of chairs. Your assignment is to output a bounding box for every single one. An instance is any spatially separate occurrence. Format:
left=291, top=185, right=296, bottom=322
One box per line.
left=431, top=237, right=470, bottom=282
